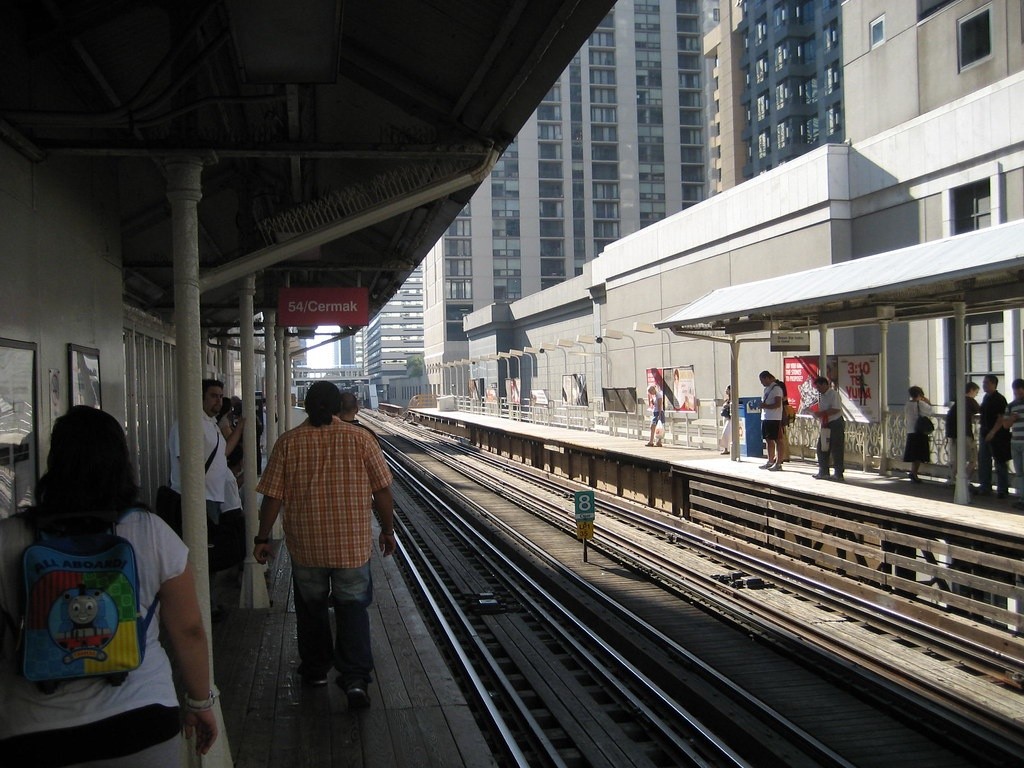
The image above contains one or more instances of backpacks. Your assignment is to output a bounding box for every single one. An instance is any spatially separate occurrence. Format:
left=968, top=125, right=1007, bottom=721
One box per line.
left=22, top=524, right=159, bottom=693
left=946, top=398, right=972, bottom=438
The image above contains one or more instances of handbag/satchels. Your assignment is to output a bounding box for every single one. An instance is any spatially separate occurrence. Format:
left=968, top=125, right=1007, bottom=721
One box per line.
left=155, top=485, right=182, bottom=539
left=821, top=410, right=830, bottom=451
left=654, top=419, right=665, bottom=439
left=915, top=399, right=934, bottom=435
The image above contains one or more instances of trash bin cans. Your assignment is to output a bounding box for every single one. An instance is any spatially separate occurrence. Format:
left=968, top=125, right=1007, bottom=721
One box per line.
left=436, top=395, right=454, bottom=411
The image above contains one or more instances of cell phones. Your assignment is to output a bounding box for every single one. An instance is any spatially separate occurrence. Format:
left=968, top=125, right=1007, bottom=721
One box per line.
left=920, top=394, right=924, bottom=398
left=1012, top=412, right=1018, bottom=417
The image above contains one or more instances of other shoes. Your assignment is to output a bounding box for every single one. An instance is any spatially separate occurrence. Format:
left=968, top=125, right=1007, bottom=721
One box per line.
left=645, top=442, right=654, bottom=446
left=908, top=473, right=922, bottom=483
left=347, top=678, right=371, bottom=707
left=306, top=670, right=327, bottom=684
left=1012, top=501, right=1024, bottom=508
left=653, top=442, right=662, bottom=447
left=768, top=464, right=782, bottom=471
left=760, top=462, right=773, bottom=468
left=995, top=493, right=1009, bottom=498
left=972, top=486, right=992, bottom=495
left=721, top=451, right=730, bottom=455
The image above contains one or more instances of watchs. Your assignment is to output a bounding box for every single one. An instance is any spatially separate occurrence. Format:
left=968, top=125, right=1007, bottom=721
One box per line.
left=254, top=536, right=269, bottom=545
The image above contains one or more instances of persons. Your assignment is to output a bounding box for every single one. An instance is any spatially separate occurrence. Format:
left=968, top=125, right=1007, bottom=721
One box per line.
left=719, top=385, right=733, bottom=455
left=644, top=386, right=666, bottom=447
left=167, top=378, right=278, bottom=653
left=252, top=379, right=396, bottom=713
left=1003, top=378, right=1024, bottom=510
left=946, top=382, right=980, bottom=488
left=973, top=374, right=1011, bottom=499
left=511, top=380, right=520, bottom=403
left=672, top=369, right=685, bottom=410
left=904, top=386, right=933, bottom=484
left=756, top=371, right=791, bottom=471
left=812, top=376, right=845, bottom=482
left=0, top=404, right=217, bottom=768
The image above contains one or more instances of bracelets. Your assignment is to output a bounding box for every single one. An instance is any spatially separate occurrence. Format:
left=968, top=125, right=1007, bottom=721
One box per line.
left=183, top=690, right=216, bottom=712
left=381, top=528, right=394, bottom=535
left=990, top=431, right=995, bottom=435
